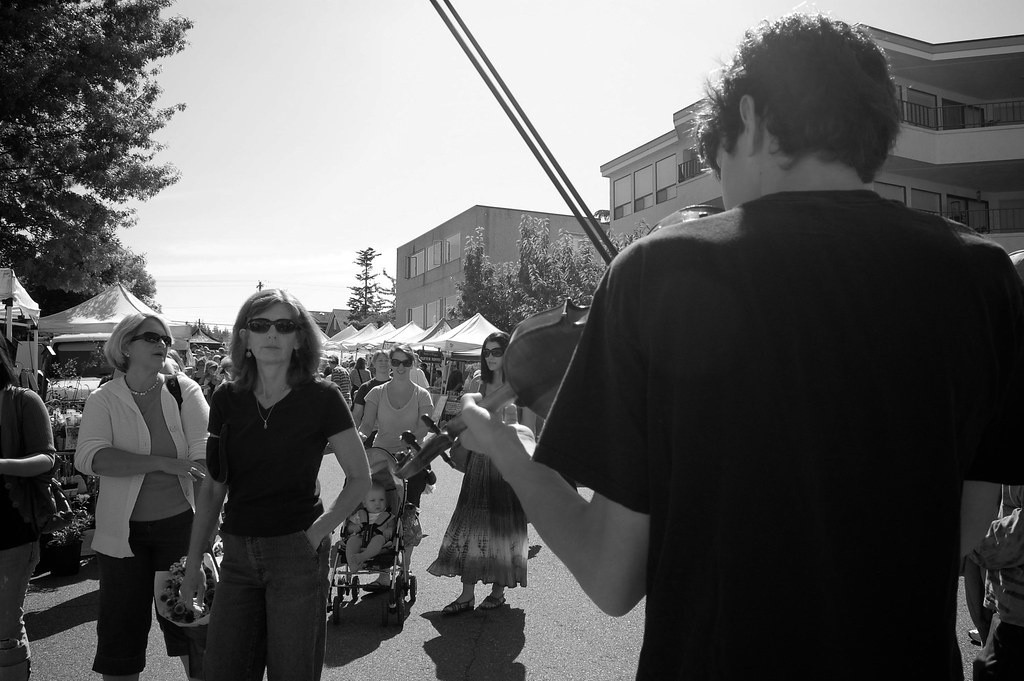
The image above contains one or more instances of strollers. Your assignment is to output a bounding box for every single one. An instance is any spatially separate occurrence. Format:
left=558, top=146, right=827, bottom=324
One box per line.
left=324, top=429, right=427, bottom=629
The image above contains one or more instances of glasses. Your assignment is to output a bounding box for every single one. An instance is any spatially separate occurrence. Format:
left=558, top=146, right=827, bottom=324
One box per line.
left=130, top=331, right=172, bottom=347
left=391, top=359, right=413, bottom=367
left=482, top=347, right=504, bottom=358
left=248, top=318, right=303, bottom=335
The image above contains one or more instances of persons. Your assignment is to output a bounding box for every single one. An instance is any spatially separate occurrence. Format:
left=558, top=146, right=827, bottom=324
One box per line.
left=73, top=313, right=205, bottom=681
left=427, top=330, right=528, bottom=614
left=162, top=334, right=446, bottom=436
left=458, top=20, right=1023, bottom=681
left=0, top=327, right=57, bottom=681
left=363, top=344, right=431, bottom=456
left=179, top=289, right=369, bottom=681
left=427, top=331, right=477, bottom=605
left=344, top=479, right=398, bottom=575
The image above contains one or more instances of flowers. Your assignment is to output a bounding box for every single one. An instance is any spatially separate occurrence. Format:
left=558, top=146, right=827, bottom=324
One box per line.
left=154, top=552, right=220, bottom=648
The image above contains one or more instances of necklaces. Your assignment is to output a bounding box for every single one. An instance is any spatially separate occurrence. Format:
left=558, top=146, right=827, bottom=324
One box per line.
left=124, top=372, right=167, bottom=398
left=252, top=381, right=282, bottom=427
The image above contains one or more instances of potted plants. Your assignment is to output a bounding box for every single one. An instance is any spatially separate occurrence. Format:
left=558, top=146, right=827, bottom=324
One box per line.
left=44, top=494, right=94, bottom=578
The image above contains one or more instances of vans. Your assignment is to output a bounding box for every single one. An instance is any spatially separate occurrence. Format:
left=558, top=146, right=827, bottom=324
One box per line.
left=38, top=332, right=123, bottom=416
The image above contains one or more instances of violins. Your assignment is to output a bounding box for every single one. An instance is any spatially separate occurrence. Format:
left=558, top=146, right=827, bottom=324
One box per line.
left=388, top=205, right=726, bottom=486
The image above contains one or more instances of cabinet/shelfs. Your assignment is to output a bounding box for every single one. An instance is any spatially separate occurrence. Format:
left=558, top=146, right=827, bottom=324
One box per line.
left=42, top=377, right=85, bottom=456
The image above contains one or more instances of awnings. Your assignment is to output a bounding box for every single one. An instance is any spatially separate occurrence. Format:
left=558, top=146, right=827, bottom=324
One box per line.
left=322, top=315, right=504, bottom=355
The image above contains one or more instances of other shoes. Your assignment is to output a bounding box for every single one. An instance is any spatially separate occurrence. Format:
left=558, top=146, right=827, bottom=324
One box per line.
left=481, top=593, right=506, bottom=610
left=396, top=576, right=404, bottom=583
left=443, top=595, right=475, bottom=615
left=363, top=581, right=391, bottom=592
